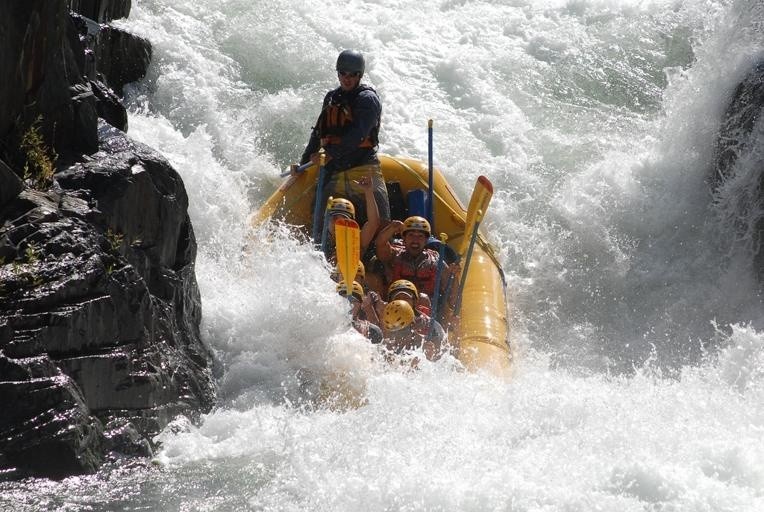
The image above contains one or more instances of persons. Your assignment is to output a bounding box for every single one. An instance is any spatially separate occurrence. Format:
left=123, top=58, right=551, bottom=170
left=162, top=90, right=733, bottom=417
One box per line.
left=319, top=175, right=464, bottom=376
left=289, top=47, right=392, bottom=246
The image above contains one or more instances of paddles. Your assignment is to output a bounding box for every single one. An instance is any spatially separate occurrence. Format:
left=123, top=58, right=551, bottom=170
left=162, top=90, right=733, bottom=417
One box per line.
left=335, top=218, right=361, bottom=302
left=438, top=175, right=494, bottom=320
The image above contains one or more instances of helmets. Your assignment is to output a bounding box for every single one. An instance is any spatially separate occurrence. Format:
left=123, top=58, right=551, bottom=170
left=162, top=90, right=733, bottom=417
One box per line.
left=336, top=280, right=364, bottom=303
left=382, top=301, right=413, bottom=333
left=337, top=50, right=366, bottom=78
left=401, top=216, right=431, bottom=239
left=324, top=198, right=355, bottom=223
left=336, top=258, right=365, bottom=278
left=387, top=279, right=419, bottom=301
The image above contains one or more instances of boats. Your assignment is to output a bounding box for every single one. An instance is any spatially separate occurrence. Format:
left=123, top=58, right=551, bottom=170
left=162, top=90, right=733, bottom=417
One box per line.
left=242, top=157, right=512, bottom=417
